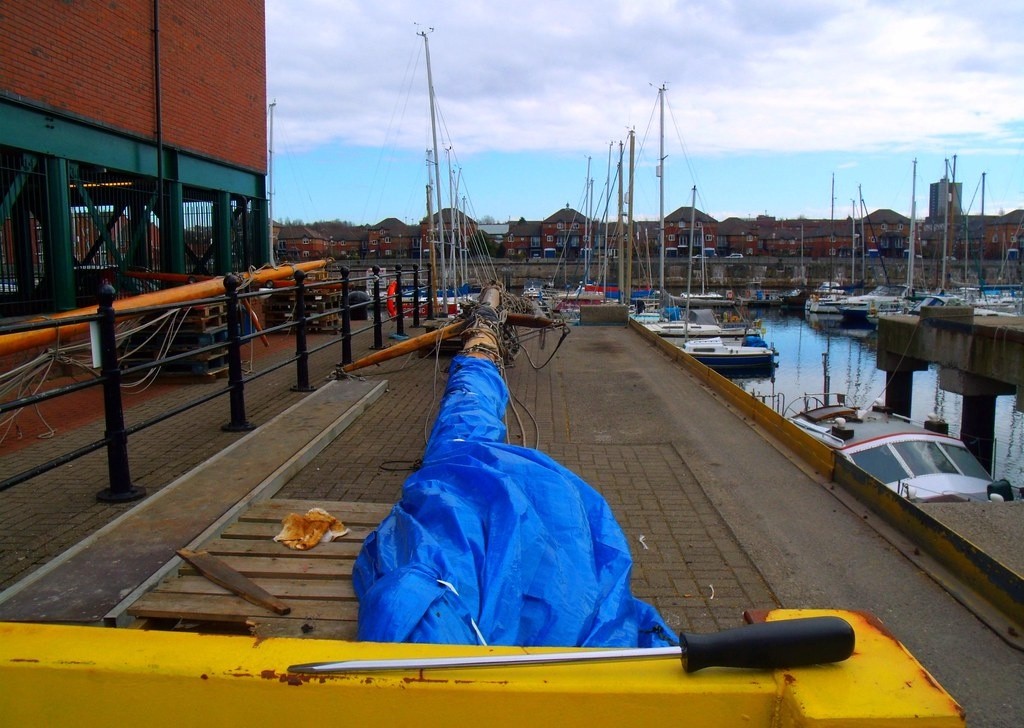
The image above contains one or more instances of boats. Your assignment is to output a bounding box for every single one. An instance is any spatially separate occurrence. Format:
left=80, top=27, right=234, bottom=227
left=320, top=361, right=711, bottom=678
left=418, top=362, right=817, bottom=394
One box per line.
left=366, top=22, right=1024, bottom=502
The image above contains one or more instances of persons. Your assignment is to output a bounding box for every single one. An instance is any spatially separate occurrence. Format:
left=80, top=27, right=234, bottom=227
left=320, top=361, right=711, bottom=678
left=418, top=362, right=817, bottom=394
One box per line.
left=635, top=297, right=646, bottom=313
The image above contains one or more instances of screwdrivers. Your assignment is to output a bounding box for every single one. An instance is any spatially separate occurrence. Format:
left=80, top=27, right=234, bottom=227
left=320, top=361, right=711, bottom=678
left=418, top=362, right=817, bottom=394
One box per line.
left=283, top=613, right=856, bottom=678
